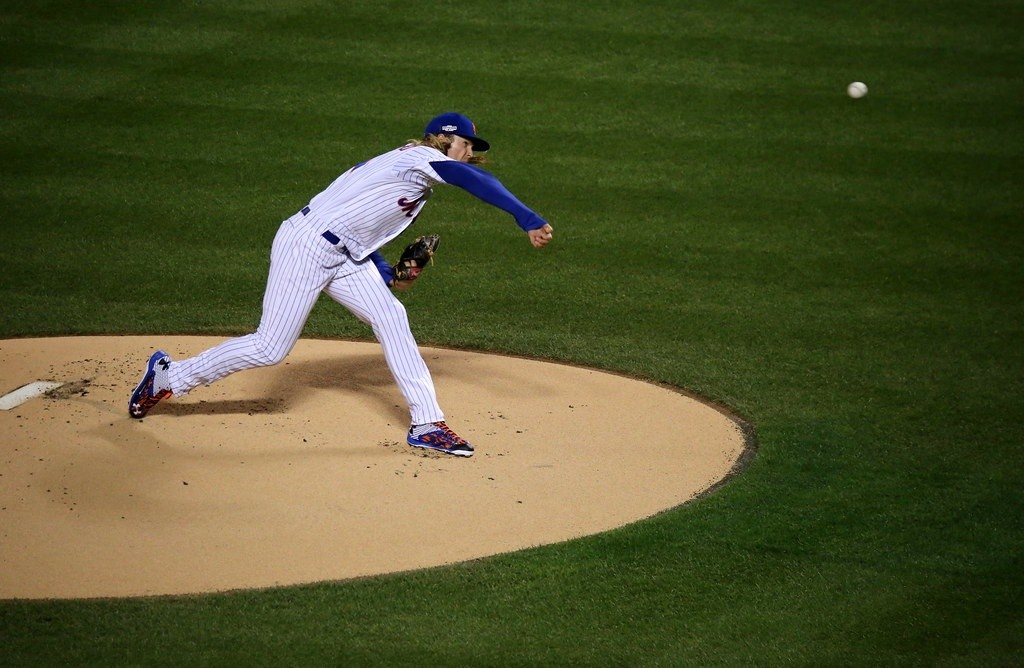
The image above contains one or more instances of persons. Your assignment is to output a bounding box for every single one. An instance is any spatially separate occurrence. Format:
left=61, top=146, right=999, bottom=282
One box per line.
left=126, top=111, right=553, bottom=458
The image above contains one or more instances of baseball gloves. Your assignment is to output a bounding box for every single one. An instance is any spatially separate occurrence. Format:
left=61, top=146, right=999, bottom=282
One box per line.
left=392, top=233, right=441, bottom=281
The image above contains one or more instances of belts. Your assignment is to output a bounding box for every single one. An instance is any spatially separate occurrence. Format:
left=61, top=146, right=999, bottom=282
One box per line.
left=301, top=206, right=350, bottom=258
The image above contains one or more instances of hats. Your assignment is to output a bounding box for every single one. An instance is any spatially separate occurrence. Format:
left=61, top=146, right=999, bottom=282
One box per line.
left=424, top=112, right=490, bottom=152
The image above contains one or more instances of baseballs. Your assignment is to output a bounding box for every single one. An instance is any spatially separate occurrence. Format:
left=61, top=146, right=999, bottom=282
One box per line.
left=846, top=81, right=868, bottom=99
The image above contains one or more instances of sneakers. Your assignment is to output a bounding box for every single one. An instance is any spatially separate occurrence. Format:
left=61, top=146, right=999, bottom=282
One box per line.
left=406, top=421, right=474, bottom=457
left=129, top=349, right=170, bottom=419
